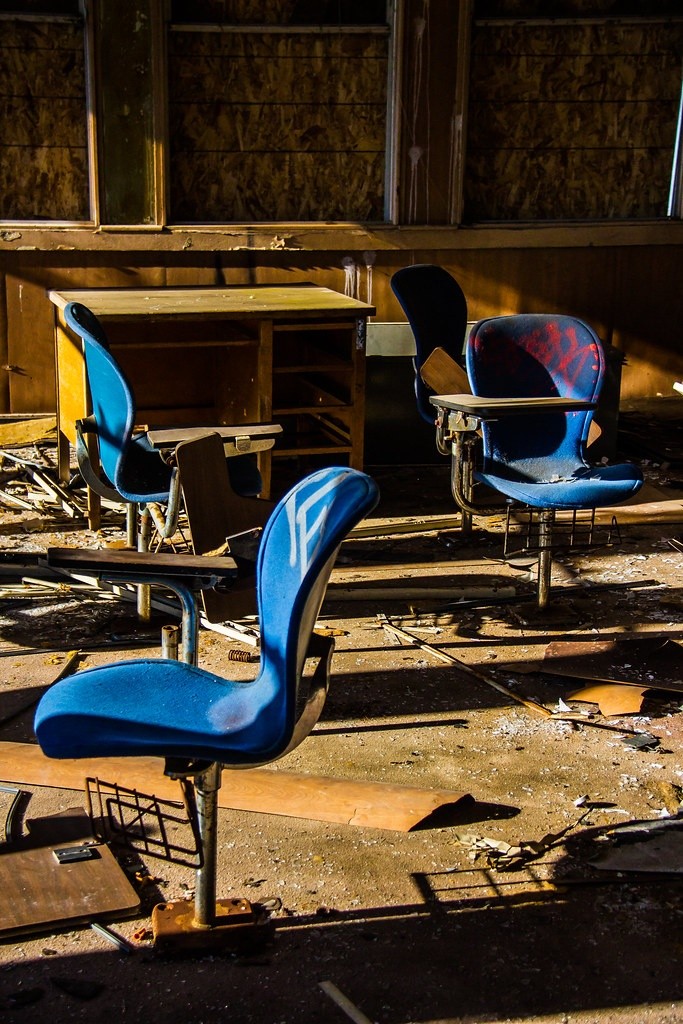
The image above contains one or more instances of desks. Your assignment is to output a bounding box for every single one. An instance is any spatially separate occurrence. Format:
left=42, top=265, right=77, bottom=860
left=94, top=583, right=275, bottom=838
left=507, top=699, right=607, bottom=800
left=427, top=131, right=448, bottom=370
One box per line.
left=46, top=282, right=378, bottom=531
left=426, top=394, right=598, bottom=517
left=46, top=546, right=238, bottom=669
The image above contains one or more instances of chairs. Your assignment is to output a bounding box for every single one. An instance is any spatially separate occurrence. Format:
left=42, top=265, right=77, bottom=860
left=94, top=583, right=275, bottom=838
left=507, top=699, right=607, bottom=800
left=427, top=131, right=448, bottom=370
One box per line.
left=34, top=466, right=378, bottom=958
left=388, top=264, right=503, bottom=551
left=464, top=313, right=646, bottom=629
left=63, top=301, right=187, bottom=644
